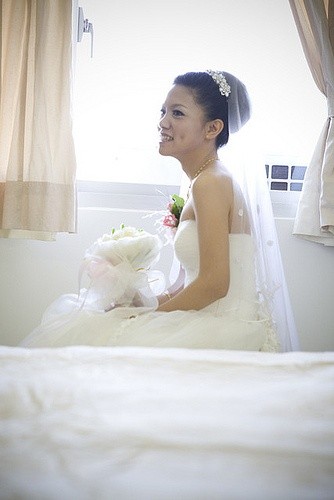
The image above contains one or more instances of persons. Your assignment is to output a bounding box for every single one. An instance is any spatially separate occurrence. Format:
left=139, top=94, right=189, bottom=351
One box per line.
left=18, top=70, right=278, bottom=352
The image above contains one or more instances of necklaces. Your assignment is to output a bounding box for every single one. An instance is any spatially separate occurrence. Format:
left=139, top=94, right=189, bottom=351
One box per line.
left=161, top=292, right=170, bottom=298
left=185, top=158, right=221, bottom=203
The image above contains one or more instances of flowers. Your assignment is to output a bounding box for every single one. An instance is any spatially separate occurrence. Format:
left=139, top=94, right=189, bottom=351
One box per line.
left=96, top=225, right=161, bottom=294
left=161, top=194, right=186, bottom=228
